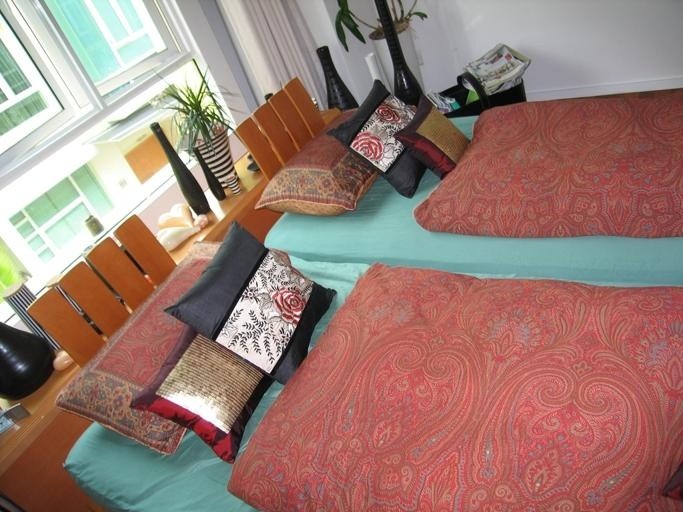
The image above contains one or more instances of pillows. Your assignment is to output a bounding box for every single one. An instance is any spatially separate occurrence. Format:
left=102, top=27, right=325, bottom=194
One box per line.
left=254, top=80, right=470, bottom=217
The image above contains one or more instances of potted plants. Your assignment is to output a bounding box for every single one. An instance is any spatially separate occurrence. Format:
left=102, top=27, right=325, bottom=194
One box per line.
left=335, top=0, right=428, bottom=108
left=148, top=67, right=245, bottom=189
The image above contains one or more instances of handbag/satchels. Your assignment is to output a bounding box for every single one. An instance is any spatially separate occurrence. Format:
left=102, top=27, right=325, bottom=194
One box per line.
left=440, top=72, right=525, bottom=117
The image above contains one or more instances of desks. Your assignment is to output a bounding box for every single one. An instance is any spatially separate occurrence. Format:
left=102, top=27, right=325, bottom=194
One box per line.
left=0, top=109, right=352, bottom=511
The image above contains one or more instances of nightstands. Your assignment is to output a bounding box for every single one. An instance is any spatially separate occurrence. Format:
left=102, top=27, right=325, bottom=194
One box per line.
left=438, top=83, right=528, bottom=118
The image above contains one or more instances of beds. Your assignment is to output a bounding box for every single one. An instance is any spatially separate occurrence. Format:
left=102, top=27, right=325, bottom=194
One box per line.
left=201, top=77, right=683, bottom=286
left=0, top=215, right=683, bottom=512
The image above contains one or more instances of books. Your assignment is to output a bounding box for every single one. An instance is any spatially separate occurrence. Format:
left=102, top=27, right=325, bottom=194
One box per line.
left=426, top=42, right=530, bottom=114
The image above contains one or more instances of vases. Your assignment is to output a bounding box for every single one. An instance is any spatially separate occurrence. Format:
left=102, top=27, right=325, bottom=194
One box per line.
left=0, top=322, right=56, bottom=400
left=149, top=122, right=210, bottom=215
left=364, top=51, right=385, bottom=87
left=316, top=46, right=359, bottom=110
left=194, top=148, right=226, bottom=201
